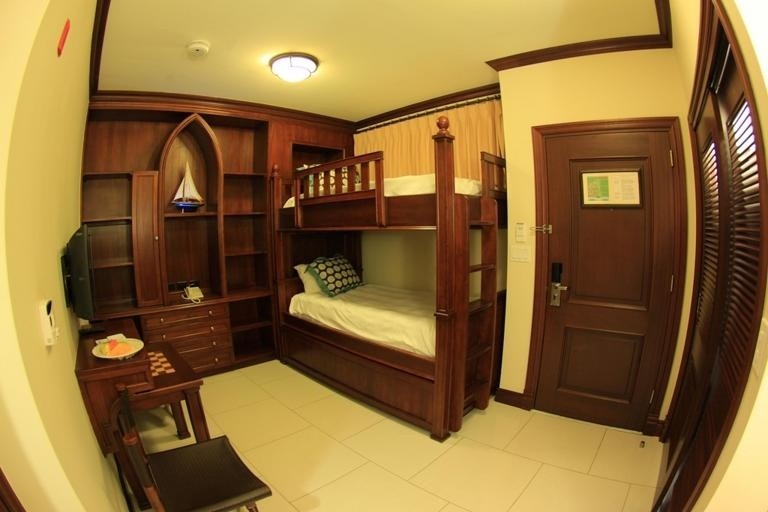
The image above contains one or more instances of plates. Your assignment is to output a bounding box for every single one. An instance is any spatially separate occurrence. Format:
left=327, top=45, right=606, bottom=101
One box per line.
left=90, top=338, right=145, bottom=362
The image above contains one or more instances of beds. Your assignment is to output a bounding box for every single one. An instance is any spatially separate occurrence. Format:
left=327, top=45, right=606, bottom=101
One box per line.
left=268, top=115, right=507, bottom=443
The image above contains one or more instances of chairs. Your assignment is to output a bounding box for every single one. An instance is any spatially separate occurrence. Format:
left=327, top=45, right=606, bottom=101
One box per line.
left=115, top=381, right=274, bottom=512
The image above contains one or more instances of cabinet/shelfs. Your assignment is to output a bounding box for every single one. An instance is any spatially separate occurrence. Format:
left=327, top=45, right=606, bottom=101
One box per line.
left=68, top=89, right=364, bottom=380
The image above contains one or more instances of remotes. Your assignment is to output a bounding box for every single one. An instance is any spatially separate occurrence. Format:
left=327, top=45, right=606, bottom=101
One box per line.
left=79, top=327, right=104, bottom=333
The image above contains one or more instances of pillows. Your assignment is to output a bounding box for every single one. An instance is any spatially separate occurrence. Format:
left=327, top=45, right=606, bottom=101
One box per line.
left=292, top=261, right=322, bottom=294
left=304, top=251, right=368, bottom=298
left=295, top=162, right=362, bottom=194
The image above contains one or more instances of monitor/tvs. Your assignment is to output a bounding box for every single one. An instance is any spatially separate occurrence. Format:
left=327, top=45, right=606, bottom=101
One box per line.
left=60, top=223, right=97, bottom=322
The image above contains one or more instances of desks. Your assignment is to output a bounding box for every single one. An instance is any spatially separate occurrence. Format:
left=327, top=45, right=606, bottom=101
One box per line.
left=75, top=318, right=212, bottom=511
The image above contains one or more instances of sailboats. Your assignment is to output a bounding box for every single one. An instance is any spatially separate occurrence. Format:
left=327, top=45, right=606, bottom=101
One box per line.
left=169, top=160, right=205, bottom=213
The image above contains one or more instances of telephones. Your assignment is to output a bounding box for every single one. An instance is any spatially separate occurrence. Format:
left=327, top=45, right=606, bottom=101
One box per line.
left=184, top=286, right=204, bottom=301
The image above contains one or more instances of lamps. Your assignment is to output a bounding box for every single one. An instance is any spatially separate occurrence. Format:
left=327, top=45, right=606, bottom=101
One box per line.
left=268, top=50, right=320, bottom=84
